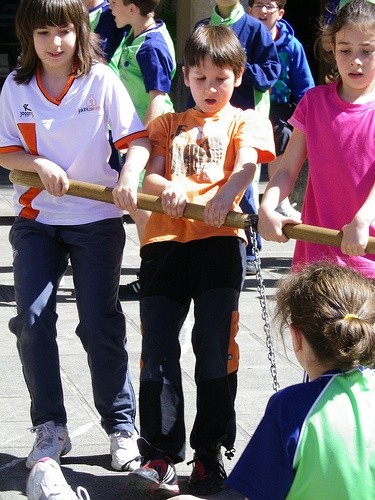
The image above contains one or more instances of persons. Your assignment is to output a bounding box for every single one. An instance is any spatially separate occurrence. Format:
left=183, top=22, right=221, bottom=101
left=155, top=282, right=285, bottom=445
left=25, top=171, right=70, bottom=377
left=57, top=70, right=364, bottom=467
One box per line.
left=26, top=257, right=375, bottom=500
left=72, top=0, right=176, bottom=302
left=247, top=0, right=316, bottom=220
left=0, top=0, right=152, bottom=472
left=127, top=25, right=276, bottom=500
left=80, top=0, right=132, bottom=64
left=186, top=0, right=281, bottom=275
left=322, top=0, right=375, bottom=86
left=257, top=0, right=375, bottom=384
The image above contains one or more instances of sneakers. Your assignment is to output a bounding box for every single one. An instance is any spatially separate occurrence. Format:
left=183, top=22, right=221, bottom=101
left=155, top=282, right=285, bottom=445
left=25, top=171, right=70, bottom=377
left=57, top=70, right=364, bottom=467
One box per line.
left=126, top=457, right=180, bottom=500
left=118, top=273, right=140, bottom=301
left=25, top=456, right=90, bottom=500
left=185, top=450, right=229, bottom=496
left=25, top=419, right=72, bottom=469
left=109, top=431, right=143, bottom=470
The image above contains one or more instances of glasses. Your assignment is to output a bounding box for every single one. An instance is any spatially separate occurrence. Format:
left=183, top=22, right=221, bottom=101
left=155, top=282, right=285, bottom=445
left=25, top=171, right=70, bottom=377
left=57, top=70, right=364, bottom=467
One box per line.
left=253, top=4, right=278, bottom=12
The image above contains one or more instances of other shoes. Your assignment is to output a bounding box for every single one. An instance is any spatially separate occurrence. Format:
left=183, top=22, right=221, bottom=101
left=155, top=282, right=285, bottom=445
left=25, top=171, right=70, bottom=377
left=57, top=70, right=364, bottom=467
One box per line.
left=245, top=258, right=259, bottom=274
